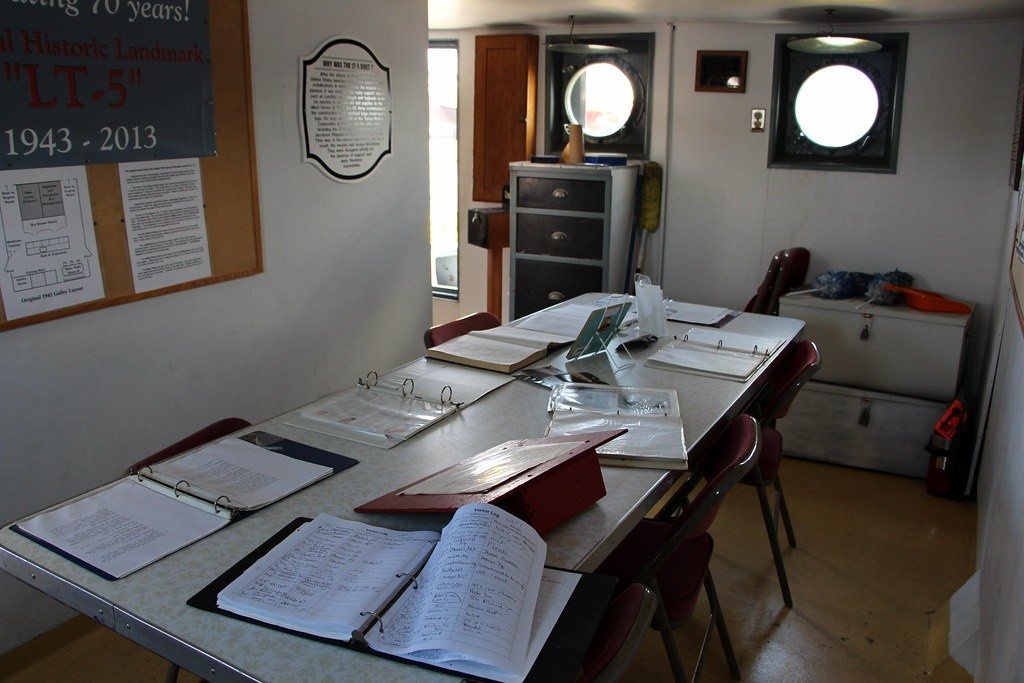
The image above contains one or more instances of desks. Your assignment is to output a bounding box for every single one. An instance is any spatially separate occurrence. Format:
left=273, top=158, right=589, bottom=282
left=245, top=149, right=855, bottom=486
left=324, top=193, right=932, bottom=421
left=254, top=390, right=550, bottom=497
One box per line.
left=0, top=291, right=806, bottom=683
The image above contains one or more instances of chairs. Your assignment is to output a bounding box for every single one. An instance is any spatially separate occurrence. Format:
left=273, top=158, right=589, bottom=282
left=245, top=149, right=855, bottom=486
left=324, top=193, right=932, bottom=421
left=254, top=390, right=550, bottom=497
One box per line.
left=582, top=340, right=821, bottom=683
left=743, top=247, right=811, bottom=315
left=423, top=313, right=501, bottom=352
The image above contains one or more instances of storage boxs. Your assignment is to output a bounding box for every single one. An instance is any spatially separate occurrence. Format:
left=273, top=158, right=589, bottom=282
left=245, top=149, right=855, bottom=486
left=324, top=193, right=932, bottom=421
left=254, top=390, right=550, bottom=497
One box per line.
left=778, top=291, right=978, bottom=402
left=776, top=380, right=948, bottom=477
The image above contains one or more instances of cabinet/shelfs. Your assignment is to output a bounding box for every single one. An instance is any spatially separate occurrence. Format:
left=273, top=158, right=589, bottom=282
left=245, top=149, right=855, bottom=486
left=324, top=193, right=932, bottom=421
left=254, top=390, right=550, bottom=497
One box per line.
left=509, top=160, right=640, bottom=323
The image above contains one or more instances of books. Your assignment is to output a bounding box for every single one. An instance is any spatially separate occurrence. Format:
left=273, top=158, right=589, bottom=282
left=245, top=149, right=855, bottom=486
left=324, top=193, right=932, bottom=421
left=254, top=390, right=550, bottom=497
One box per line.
left=425, top=325, right=577, bottom=374
left=9, top=431, right=361, bottom=582
left=283, top=358, right=515, bottom=450
left=643, top=326, right=787, bottom=382
left=184, top=515, right=590, bottom=683
left=547, top=380, right=688, bottom=471
